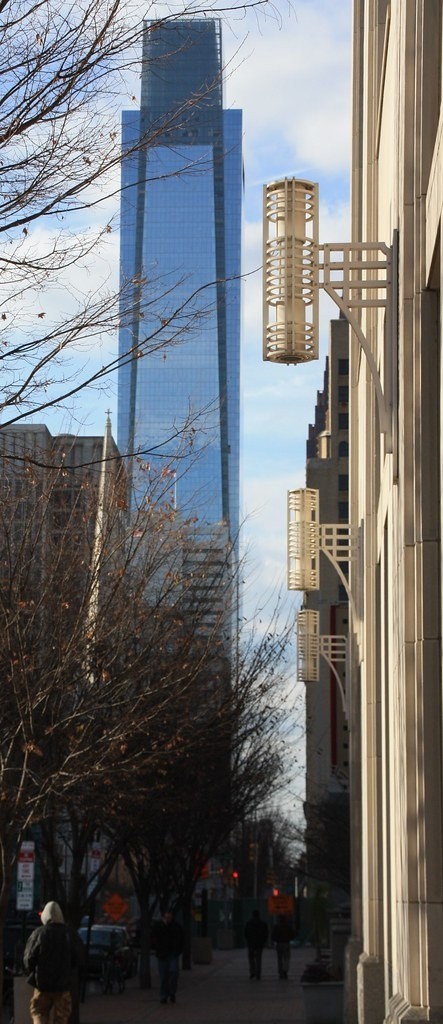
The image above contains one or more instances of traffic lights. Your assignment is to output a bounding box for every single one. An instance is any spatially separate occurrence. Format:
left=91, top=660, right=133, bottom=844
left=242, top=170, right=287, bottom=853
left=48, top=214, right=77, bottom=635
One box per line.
left=233, top=871, right=240, bottom=888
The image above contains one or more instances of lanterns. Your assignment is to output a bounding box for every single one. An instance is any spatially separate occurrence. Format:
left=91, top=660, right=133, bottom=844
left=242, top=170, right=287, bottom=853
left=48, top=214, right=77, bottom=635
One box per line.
left=218, top=867, right=239, bottom=879
left=272, top=888, right=279, bottom=898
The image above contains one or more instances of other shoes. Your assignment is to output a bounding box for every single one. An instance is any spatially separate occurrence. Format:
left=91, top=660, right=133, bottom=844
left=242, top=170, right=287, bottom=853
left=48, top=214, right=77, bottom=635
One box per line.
left=255, top=971, right=260, bottom=978
left=249, top=971, right=256, bottom=979
left=161, top=999, right=168, bottom=1004
left=170, top=996, right=176, bottom=1003
left=280, top=973, right=287, bottom=980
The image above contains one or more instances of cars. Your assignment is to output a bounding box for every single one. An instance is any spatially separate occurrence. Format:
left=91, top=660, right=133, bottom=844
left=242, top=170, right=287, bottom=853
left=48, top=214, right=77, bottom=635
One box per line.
left=74, top=923, right=134, bottom=978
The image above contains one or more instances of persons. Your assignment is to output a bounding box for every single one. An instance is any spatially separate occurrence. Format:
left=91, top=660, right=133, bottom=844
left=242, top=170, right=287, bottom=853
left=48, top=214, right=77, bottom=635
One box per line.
left=244, top=910, right=296, bottom=981
left=22, top=900, right=86, bottom=1024
left=149, top=908, right=184, bottom=1005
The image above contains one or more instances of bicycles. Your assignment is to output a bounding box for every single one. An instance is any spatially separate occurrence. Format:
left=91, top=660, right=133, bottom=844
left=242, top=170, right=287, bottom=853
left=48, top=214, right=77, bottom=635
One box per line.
left=99, top=950, right=127, bottom=995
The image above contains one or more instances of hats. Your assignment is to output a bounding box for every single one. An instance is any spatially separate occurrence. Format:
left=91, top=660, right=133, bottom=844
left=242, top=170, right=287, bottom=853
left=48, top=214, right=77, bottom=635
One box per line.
left=40, top=901, right=65, bottom=926
